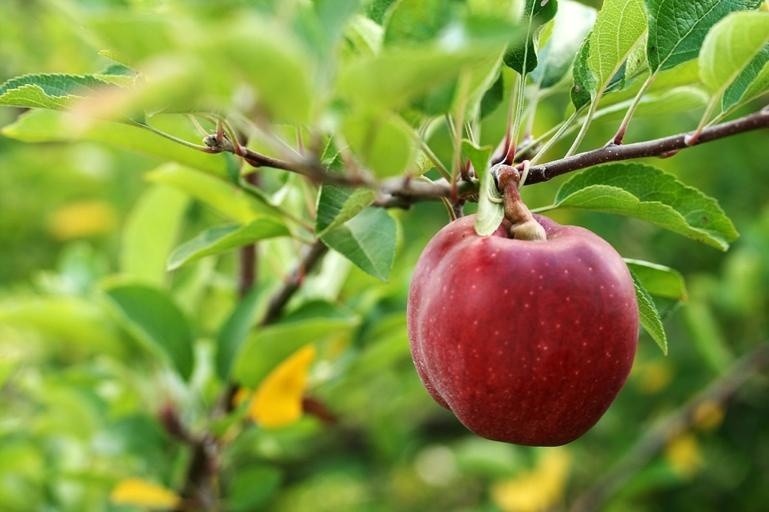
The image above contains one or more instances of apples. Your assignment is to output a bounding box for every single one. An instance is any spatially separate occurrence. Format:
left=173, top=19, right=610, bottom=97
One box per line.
left=407, top=212, right=639, bottom=446
